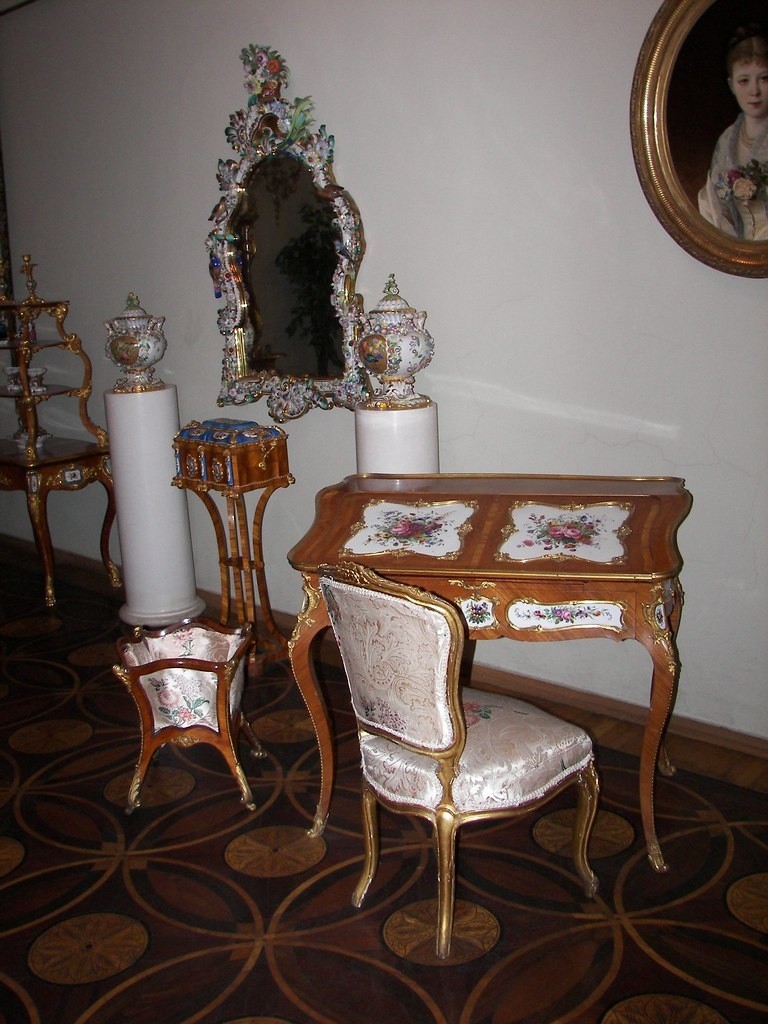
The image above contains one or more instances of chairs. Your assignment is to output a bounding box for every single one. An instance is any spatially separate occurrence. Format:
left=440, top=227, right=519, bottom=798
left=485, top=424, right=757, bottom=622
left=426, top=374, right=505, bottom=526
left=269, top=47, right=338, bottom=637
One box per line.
left=320, top=559, right=601, bottom=959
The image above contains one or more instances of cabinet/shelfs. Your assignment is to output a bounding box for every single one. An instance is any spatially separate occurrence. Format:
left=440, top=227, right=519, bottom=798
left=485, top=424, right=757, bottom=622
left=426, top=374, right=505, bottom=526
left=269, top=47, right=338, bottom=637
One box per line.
left=0, top=299, right=124, bottom=607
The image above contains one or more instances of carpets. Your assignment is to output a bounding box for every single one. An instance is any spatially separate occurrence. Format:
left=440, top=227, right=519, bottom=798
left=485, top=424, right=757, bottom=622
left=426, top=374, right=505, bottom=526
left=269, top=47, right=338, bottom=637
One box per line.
left=0, top=560, right=768, bottom=1024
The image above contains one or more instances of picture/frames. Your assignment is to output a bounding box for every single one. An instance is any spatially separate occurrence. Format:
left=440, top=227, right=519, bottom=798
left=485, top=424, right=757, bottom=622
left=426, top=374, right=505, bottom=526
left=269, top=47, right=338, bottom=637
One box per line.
left=629, top=0, right=768, bottom=281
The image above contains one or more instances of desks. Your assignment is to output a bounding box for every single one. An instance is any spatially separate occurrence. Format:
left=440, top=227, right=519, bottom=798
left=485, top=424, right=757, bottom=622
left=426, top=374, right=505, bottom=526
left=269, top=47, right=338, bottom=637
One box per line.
left=286, top=472, right=694, bottom=878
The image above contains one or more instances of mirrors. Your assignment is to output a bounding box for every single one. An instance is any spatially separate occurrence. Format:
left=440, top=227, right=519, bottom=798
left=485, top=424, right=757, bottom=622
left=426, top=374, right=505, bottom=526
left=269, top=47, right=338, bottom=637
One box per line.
left=204, top=41, right=367, bottom=422
